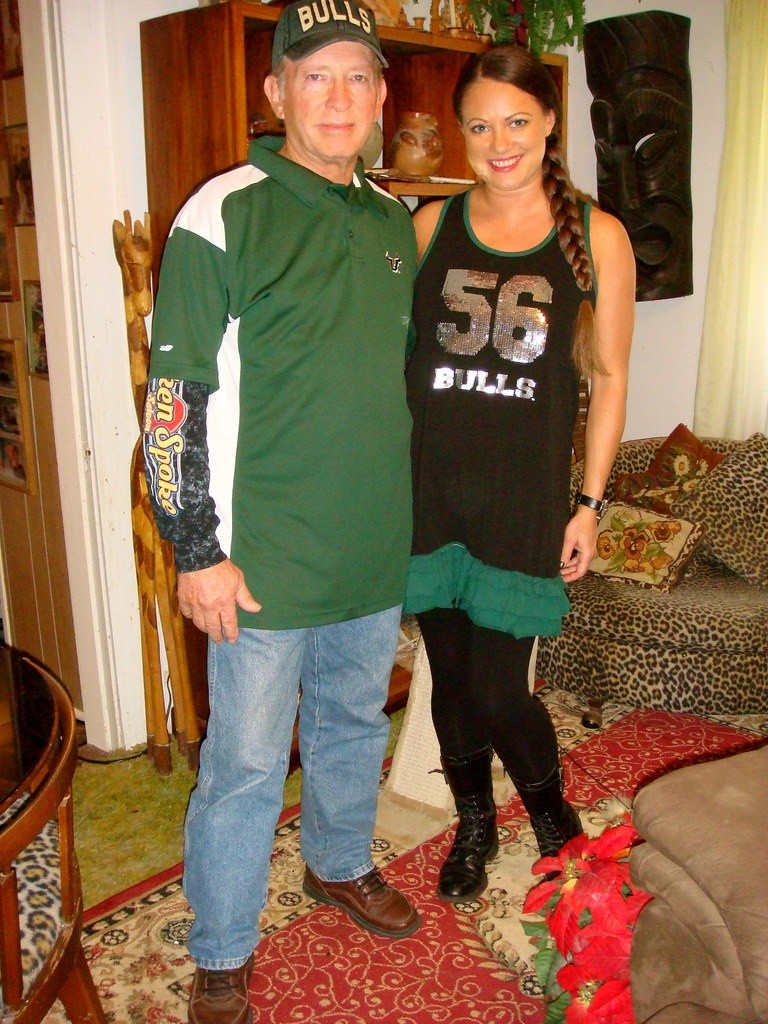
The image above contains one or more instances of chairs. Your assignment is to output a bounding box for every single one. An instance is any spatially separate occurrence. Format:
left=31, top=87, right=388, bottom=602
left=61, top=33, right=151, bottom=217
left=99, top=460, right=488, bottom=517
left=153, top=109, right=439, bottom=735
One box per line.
left=0, top=657, right=108, bottom=1024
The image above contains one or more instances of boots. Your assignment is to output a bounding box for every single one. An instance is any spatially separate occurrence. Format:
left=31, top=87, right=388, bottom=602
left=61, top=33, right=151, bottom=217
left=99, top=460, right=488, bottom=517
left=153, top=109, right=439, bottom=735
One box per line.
left=428, top=745, right=499, bottom=902
left=504, top=763, right=583, bottom=881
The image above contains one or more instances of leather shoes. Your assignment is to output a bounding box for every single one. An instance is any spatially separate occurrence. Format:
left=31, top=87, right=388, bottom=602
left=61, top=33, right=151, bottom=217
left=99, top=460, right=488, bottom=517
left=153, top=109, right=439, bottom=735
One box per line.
left=302, top=863, right=421, bottom=937
left=188, top=951, right=255, bottom=1024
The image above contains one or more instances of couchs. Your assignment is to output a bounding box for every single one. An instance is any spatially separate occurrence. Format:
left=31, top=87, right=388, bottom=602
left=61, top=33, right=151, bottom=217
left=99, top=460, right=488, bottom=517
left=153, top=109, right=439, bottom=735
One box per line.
left=538, top=436, right=768, bottom=729
left=628, top=743, right=767, bottom=1024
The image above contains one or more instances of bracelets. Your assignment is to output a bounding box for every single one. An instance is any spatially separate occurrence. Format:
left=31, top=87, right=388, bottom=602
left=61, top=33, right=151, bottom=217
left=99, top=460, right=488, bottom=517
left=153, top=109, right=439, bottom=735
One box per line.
left=575, top=493, right=610, bottom=520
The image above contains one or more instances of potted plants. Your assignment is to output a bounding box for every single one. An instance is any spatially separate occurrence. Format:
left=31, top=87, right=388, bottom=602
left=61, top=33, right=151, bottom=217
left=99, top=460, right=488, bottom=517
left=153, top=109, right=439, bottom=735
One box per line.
left=465, top=0, right=586, bottom=59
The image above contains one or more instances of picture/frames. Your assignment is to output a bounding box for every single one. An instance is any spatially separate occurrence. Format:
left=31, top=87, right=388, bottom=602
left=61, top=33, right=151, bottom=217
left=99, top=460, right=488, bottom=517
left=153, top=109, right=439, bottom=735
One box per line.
left=21, top=278, right=49, bottom=380
left=1, top=335, right=40, bottom=498
left=0, top=196, right=22, bottom=303
left=0, top=0, right=23, bottom=79
left=5, top=123, right=34, bottom=227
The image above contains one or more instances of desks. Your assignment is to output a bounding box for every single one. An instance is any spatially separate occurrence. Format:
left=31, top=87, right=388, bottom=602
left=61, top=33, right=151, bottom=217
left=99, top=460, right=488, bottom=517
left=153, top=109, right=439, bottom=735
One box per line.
left=0, top=643, right=57, bottom=814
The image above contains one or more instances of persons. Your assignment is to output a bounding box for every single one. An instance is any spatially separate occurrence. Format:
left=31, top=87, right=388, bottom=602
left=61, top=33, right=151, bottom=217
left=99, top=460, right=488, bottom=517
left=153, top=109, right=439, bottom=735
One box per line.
left=143, top=0, right=423, bottom=1021
left=409, top=41, right=636, bottom=901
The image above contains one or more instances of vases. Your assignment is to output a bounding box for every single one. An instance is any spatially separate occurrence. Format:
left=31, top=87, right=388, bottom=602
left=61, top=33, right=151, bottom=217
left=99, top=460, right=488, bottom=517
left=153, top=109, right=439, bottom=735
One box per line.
left=388, top=110, right=442, bottom=177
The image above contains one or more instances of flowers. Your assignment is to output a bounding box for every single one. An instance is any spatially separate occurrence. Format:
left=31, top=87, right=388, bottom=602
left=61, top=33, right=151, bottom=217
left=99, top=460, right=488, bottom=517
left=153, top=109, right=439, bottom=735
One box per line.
left=522, top=814, right=654, bottom=1024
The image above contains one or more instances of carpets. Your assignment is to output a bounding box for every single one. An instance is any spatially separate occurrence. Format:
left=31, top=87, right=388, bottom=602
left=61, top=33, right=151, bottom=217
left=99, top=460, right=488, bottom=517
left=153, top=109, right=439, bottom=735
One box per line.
left=40, top=676, right=767, bottom=1024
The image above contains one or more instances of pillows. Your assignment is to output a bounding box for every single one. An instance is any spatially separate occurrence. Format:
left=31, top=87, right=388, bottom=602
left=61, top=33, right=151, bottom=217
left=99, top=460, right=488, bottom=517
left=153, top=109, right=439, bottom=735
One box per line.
left=669, top=431, right=768, bottom=590
left=587, top=497, right=704, bottom=595
left=616, top=423, right=725, bottom=520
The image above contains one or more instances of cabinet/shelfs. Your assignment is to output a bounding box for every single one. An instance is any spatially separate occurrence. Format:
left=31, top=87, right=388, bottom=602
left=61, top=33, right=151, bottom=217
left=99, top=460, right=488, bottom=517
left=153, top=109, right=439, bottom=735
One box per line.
left=138, top=0, right=568, bottom=790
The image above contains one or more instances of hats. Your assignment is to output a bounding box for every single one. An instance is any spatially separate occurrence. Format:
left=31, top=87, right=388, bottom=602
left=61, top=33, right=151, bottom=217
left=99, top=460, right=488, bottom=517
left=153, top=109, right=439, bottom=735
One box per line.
left=271, top=0, right=389, bottom=70
